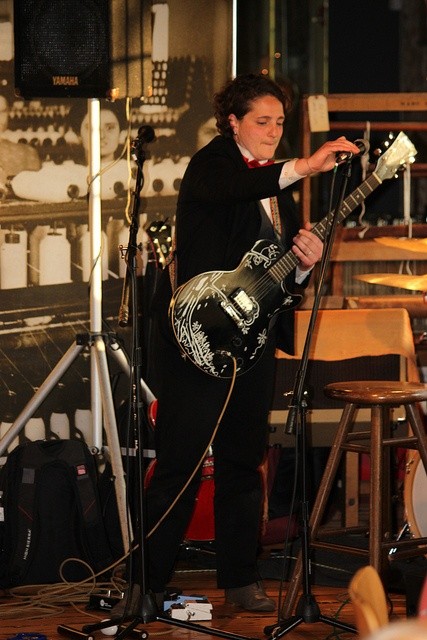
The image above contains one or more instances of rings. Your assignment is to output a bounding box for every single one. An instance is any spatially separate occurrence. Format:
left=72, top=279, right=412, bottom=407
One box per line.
left=307, top=251, right=313, bottom=256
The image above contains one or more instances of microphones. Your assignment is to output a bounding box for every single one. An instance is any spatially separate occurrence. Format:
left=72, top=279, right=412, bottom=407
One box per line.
left=337, top=138, right=369, bottom=162
left=130, top=126, right=155, bottom=152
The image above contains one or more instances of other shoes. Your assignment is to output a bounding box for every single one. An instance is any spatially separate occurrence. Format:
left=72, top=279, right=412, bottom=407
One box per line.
left=108, top=583, right=168, bottom=618
left=225, top=581, right=276, bottom=611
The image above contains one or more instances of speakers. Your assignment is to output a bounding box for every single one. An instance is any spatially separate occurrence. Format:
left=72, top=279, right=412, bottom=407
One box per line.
left=150, top=445, right=271, bottom=544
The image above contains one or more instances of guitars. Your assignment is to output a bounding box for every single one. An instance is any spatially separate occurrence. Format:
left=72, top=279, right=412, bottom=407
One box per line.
left=168, top=129, right=419, bottom=379
left=143, top=218, right=270, bottom=542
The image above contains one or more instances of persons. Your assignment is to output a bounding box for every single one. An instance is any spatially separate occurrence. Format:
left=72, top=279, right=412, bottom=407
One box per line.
left=110, top=76, right=359, bottom=615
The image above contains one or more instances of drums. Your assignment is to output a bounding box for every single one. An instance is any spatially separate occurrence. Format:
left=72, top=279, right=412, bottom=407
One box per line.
left=404, top=449, right=427, bottom=559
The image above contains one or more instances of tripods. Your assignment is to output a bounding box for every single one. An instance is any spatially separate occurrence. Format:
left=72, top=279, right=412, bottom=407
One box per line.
left=87, top=155, right=359, bottom=640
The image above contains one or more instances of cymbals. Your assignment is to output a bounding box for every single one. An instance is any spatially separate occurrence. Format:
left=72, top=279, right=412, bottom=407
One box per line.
left=373, top=237, right=427, bottom=254
left=352, top=273, right=427, bottom=291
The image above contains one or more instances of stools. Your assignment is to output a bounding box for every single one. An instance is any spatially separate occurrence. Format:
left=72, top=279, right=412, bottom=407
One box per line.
left=281, top=381, right=427, bottom=620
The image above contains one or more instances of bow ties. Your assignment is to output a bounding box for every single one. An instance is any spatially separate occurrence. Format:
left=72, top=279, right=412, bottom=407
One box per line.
left=243, top=156, right=275, bottom=169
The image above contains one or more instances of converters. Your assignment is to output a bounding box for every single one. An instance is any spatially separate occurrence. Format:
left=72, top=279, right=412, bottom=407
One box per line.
left=91, top=593, right=123, bottom=609
